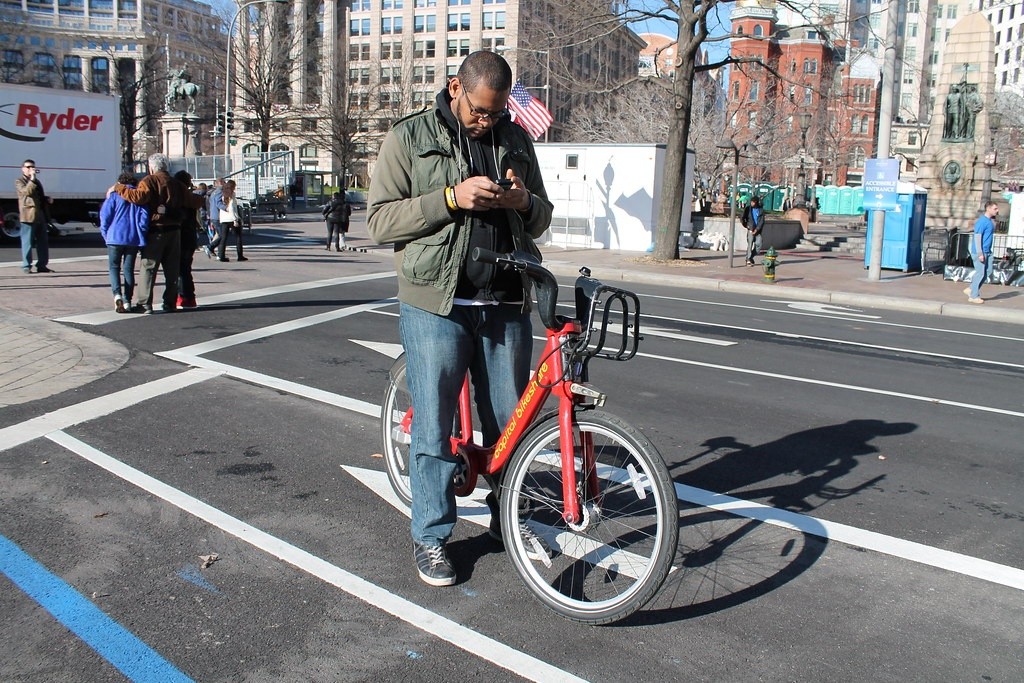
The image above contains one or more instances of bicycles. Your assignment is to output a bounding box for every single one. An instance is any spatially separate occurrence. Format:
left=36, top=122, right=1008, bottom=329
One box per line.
left=381, top=247, right=680, bottom=626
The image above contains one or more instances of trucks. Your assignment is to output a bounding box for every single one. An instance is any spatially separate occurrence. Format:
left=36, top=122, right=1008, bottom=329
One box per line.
left=0, top=81, right=124, bottom=245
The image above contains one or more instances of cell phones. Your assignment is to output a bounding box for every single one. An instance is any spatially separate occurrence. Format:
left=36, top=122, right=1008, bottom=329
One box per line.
left=493, top=179, right=513, bottom=191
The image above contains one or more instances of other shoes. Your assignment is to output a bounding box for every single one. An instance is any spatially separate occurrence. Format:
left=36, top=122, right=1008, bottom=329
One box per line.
left=968, top=296, right=984, bottom=303
left=24, top=269, right=32, bottom=274
left=964, top=287, right=971, bottom=295
left=216, top=256, right=221, bottom=260
left=131, top=304, right=153, bottom=313
left=237, top=257, right=248, bottom=261
left=745, top=257, right=754, bottom=266
left=114, top=295, right=125, bottom=313
left=325, top=247, right=330, bottom=250
left=203, top=245, right=210, bottom=258
left=124, top=302, right=131, bottom=312
left=37, top=267, right=54, bottom=273
left=220, top=258, right=229, bottom=262
left=337, top=248, right=343, bottom=252
left=162, top=304, right=175, bottom=311
left=208, top=245, right=216, bottom=256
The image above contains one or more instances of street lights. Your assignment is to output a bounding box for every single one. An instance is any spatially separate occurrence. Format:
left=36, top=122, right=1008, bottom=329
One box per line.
left=976, top=98, right=1004, bottom=216
left=224, top=0, right=289, bottom=182
left=796, top=108, right=812, bottom=202
left=716, top=140, right=749, bottom=270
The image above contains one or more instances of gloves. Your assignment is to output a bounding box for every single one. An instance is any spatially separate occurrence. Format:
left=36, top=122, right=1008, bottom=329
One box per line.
left=157, top=204, right=166, bottom=214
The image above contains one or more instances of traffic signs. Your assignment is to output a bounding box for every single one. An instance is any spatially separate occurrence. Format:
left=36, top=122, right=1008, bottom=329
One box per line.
left=863, top=158, right=899, bottom=210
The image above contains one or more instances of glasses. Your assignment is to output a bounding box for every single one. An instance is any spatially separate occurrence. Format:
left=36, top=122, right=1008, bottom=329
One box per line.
left=24, top=166, right=35, bottom=169
left=461, top=81, right=509, bottom=120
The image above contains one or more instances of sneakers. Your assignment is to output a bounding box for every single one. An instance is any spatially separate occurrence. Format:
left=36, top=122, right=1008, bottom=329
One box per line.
left=489, top=517, right=552, bottom=560
left=175, top=294, right=197, bottom=309
left=411, top=540, right=456, bottom=587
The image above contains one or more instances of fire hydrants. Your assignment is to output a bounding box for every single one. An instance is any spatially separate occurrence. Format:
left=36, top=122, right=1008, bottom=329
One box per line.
left=761, top=246, right=779, bottom=280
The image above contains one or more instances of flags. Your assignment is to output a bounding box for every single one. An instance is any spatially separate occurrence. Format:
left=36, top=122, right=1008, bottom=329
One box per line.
left=505, top=82, right=553, bottom=142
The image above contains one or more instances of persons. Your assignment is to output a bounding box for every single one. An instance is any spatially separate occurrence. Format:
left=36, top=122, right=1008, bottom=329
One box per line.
left=782, top=198, right=796, bottom=212
left=945, top=164, right=959, bottom=183
left=15, top=159, right=54, bottom=273
left=947, top=84, right=983, bottom=139
left=692, top=187, right=740, bottom=212
left=189, top=177, right=248, bottom=262
left=323, top=192, right=351, bottom=252
left=740, top=196, right=764, bottom=266
left=367, top=50, right=555, bottom=585
left=99, top=153, right=203, bottom=314
left=963, top=200, right=998, bottom=304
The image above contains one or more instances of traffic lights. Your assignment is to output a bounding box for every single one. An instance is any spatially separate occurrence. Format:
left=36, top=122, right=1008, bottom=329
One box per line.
left=227, top=111, right=235, bottom=129
left=218, top=113, right=224, bottom=133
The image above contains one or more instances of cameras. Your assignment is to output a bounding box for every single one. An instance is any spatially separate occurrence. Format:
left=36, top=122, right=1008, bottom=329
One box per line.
left=35, top=170, right=40, bottom=173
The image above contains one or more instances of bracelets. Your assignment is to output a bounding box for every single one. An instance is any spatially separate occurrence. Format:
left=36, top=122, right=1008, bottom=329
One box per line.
left=444, top=185, right=459, bottom=211
